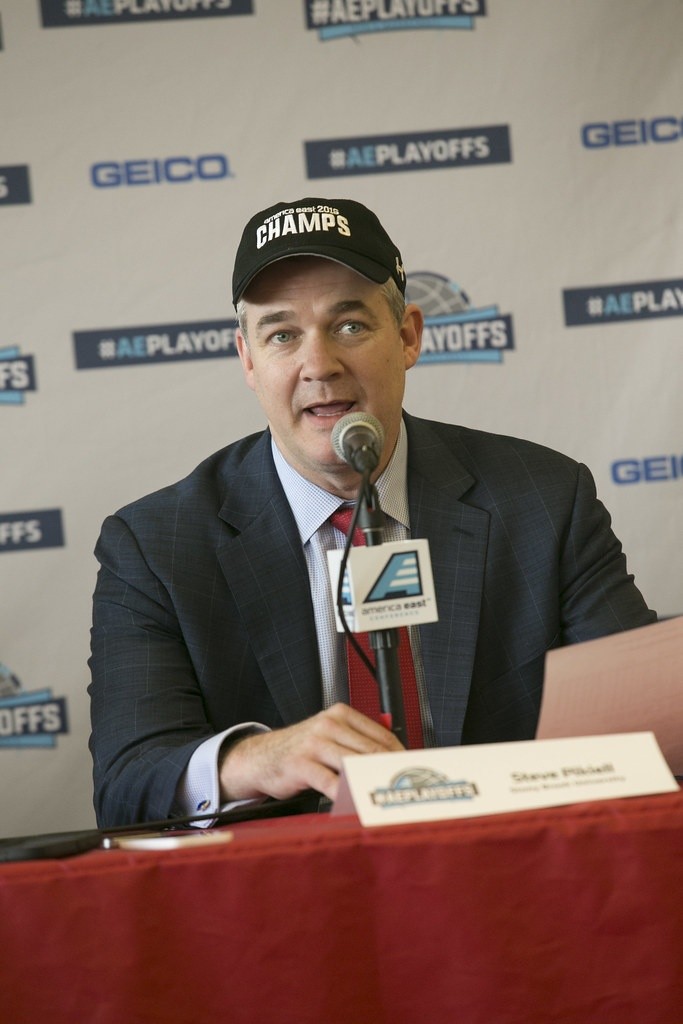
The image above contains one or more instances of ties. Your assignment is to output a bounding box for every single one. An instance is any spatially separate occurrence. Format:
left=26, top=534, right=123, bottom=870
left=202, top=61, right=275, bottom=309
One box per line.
left=330, top=506, right=424, bottom=751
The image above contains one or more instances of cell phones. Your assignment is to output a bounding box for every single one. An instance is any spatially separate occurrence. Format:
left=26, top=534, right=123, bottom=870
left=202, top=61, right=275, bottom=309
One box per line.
left=0, top=831, right=105, bottom=863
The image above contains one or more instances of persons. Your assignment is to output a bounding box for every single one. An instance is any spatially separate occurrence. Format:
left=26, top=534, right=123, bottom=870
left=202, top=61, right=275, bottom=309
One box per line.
left=87, top=196, right=656, bottom=831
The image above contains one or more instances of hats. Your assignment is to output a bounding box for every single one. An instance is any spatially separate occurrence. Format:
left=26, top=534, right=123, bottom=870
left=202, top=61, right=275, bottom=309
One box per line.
left=232, top=197, right=406, bottom=313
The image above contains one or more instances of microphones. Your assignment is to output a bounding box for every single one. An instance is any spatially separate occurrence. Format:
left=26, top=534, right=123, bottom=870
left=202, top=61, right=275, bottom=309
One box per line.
left=330, top=412, right=384, bottom=475
